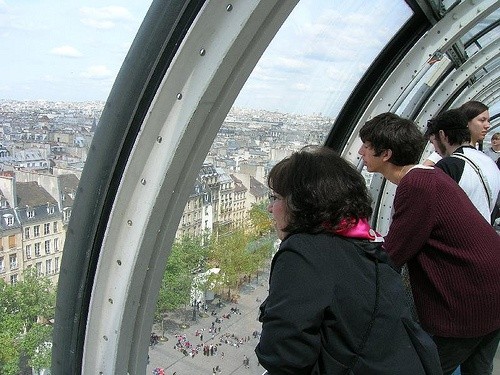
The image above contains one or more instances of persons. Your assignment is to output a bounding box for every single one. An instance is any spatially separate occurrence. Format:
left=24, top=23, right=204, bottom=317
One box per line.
left=420, top=100, right=500, bottom=233
left=254, top=143, right=443, bottom=375
left=357, top=112, right=500, bottom=375
left=145, top=298, right=264, bottom=375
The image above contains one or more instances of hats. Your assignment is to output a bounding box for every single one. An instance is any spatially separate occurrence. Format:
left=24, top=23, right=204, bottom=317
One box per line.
left=424, top=108, right=469, bottom=134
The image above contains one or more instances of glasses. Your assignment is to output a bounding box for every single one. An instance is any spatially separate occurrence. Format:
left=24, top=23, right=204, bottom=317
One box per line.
left=268, top=191, right=283, bottom=203
